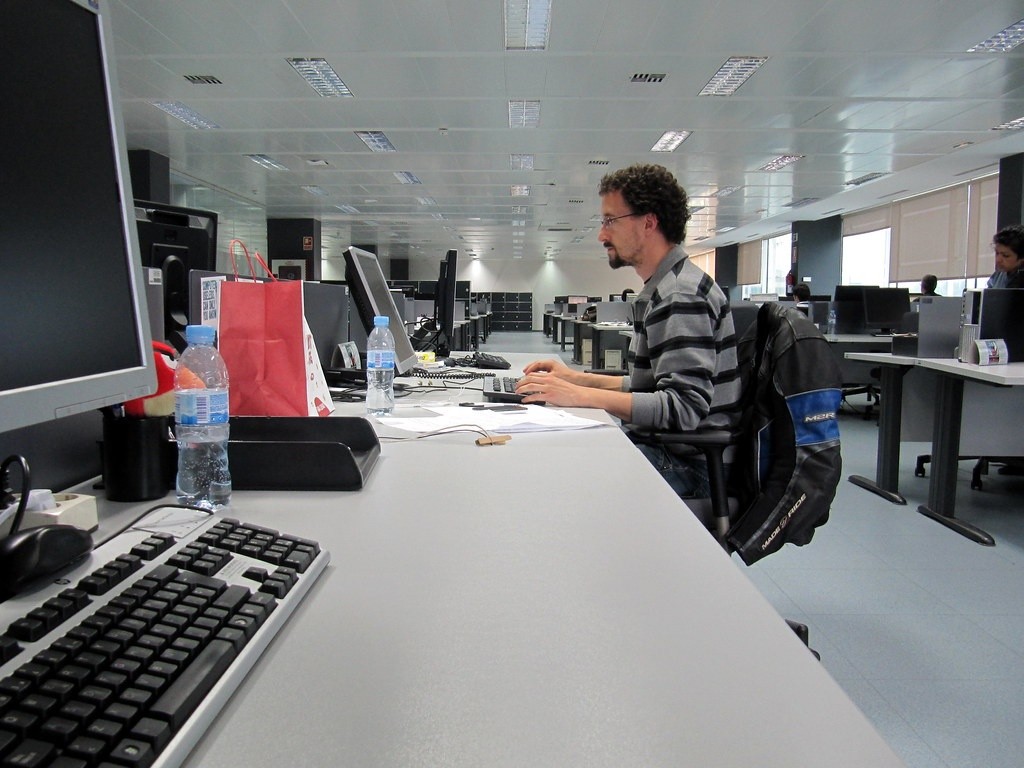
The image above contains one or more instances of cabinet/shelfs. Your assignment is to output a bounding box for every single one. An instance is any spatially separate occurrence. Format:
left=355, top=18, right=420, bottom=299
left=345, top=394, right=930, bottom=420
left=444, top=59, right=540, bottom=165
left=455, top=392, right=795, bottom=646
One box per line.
left=471, top=292, right=533, bottom=332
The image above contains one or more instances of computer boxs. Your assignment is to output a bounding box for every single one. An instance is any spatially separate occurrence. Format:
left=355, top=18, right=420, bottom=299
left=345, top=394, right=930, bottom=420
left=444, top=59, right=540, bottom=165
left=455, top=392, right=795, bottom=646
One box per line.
left=582, top=339, right=593, bottom=365
left=605, top=350, right=623, bottom=371
left=960, top=289, right=981, bottom=329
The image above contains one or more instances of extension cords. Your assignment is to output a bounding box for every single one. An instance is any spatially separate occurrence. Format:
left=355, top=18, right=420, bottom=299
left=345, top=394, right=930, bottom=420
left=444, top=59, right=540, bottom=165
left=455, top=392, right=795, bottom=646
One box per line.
left=0, top=493, right=99, bottom=540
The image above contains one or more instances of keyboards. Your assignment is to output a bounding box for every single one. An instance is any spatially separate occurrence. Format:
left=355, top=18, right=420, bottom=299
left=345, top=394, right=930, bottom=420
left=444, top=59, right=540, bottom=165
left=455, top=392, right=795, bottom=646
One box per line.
left=0, top=506, right=330, bottom=768
left=483, top=376, right=545, bottom=406
left=473, top=351, right=511, bottom=369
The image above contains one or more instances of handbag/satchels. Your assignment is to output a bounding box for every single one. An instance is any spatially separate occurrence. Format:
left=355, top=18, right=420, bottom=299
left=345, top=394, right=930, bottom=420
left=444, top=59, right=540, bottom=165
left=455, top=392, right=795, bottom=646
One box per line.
left=201, top=237, right=336, bottom=417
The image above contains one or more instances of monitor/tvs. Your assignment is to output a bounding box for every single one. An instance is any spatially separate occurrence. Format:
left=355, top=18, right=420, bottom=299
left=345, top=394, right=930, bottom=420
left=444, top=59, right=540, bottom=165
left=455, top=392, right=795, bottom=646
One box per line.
left=436, top=249, right=457, bottom=337
left=750, top=293, right=832, bottom=309
left=554, top=293, right=639, bottom=313
left=132, top=195, right=219, bottom=346
left=833, top=284, right=911, bottom=336
left=0, top=0, right=158, bottom=434
left=343, top=246, right=418, bottom=390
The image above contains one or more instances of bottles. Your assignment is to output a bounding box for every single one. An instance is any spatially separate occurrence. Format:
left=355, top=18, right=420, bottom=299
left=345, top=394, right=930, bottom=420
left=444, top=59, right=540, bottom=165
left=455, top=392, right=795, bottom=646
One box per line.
left=366, top=316, right=396, bottom=417
left=827, top=310, right=836, bottom=339
left=175, top=325, right=231, bottom=512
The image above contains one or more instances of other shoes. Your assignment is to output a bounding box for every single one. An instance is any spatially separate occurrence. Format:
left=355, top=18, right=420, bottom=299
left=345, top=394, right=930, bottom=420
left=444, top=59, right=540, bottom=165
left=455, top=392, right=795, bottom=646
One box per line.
left=998, top=466, right=1024, bottom=476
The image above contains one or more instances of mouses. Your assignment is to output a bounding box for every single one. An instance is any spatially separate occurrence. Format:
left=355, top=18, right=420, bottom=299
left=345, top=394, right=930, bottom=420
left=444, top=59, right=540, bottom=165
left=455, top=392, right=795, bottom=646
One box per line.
left=0, top=524, right=93, bottom=604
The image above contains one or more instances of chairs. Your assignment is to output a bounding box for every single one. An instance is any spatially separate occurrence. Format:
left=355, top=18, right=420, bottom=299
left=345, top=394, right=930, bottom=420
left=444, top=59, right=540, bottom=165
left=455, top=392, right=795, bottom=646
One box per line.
left=583, top=301, right=844, bottom=664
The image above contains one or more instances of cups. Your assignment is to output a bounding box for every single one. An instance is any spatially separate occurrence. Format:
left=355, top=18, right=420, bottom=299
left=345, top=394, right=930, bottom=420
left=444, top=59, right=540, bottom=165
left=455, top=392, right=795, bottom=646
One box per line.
left=102, top=416, right=177, bottom=502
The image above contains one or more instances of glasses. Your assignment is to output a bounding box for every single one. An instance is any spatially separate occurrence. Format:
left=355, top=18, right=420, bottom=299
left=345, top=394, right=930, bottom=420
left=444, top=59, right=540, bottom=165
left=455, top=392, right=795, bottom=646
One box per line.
left=601, top=210, right=659, bottom=230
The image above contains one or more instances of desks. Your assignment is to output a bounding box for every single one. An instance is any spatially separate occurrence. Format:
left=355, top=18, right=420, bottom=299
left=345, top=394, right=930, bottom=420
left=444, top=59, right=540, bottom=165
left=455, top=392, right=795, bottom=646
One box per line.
left=0, top=289, right=1024, bottom=768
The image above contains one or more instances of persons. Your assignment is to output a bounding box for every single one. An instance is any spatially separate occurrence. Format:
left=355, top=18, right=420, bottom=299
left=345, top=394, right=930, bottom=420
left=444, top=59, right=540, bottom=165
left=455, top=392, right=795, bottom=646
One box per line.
left=913, top=274, right=942, bottom=302
left=792, top=283, right=810, bottom=302
left=515, top=163, right=743, bottom=498
left=984, top=224, right=1024, bottom=472
